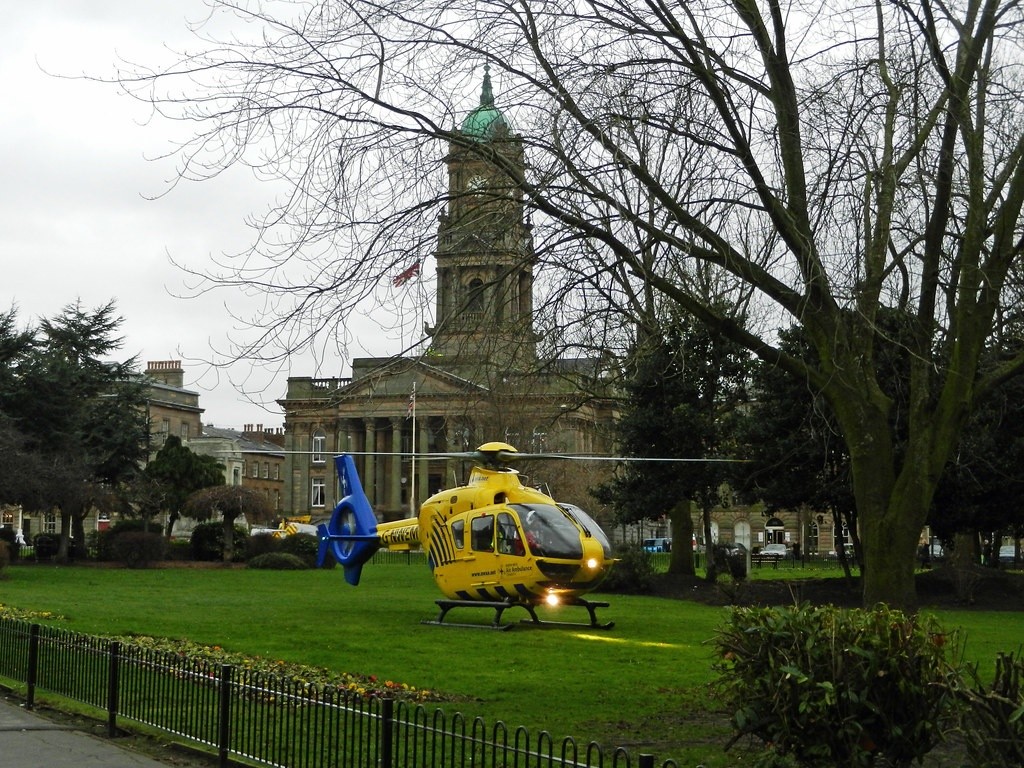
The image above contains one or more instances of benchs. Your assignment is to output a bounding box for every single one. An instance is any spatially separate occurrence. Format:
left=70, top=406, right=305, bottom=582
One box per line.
left=751, top=554, right=784, bottom=569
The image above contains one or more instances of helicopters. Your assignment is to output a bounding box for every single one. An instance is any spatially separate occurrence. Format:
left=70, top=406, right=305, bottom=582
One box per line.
left=312, top=439, right=759, bottom=631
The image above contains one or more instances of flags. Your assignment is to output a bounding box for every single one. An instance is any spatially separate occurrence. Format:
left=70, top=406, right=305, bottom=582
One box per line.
left=393, top=259, right=419, bottom=288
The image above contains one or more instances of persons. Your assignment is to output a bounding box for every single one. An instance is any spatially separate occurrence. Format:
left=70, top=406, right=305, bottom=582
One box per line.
left=524, top=510, right=553, bottom=557
left=792, top=539, right=801, bottom=560
left=665, top=537, right=672, bottom=553
left=982, top=539, right=992, bottom=567
left=918, top=539, right=932, bottom=572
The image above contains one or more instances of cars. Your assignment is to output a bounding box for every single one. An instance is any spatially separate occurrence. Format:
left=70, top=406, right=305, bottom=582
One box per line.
left=760, top=542, right=788, bottom=561
left=637, top=533, right=751, bottom=559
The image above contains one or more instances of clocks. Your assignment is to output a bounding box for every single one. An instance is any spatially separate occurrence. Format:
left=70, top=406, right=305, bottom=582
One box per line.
left=466, top=174, right=489, bottom=198
